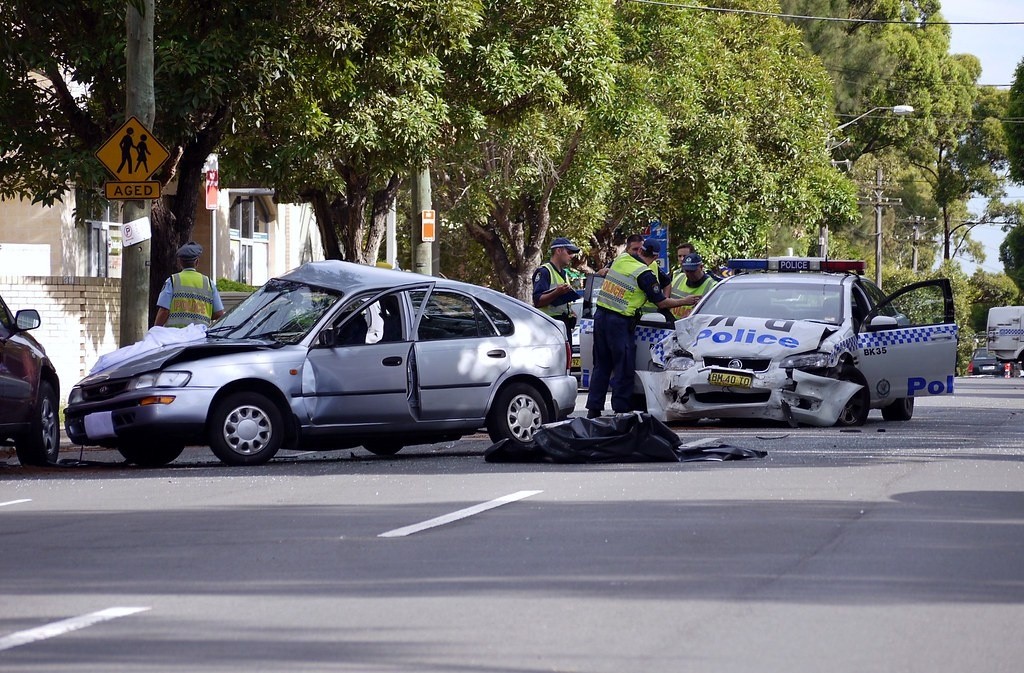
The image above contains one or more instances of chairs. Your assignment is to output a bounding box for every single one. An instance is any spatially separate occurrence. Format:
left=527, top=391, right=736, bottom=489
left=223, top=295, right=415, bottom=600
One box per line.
left=822, top=297, right=850, bottom=324
left=332, top=311, right=365, bottom=347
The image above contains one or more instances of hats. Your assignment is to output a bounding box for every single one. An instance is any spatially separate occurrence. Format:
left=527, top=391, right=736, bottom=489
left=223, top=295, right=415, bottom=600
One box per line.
left=551, top=238, right=580, bottom=253
left=175, top=241, right=203, bottom=260
left=641, top=238, right=661, bottom=255
left=681, top=254, right=702, bottom=270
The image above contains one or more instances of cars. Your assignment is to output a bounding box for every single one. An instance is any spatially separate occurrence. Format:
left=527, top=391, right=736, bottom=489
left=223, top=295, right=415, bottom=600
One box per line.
left=580, top=257, right=960, bottom=429
left=0, top=296, right=60, bottom=470
left=968, top=348, right=1014, bottom=376
left=64, top=260, right=578, bottom=466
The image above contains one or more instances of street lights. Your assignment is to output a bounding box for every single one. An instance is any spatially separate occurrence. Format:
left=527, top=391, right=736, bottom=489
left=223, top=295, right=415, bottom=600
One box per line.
left=949, top=222, right=976, bottom=236
left=829, top=105, right=915, bottom=133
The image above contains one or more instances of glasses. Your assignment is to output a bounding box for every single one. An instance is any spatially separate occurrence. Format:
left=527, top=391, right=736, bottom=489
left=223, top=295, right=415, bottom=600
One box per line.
left=679, top=255, right=686, bottom=257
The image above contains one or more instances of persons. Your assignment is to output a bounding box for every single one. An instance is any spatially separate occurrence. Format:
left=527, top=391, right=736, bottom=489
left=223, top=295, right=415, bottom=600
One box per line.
left=668, top=253, right=718, bottom=322
left=531, top=238, right=581, bottom=354
left=153, top=241, right=224, bottom=328
left=596, top=234, right=671, bottom=315
left=585, top=239, right=702, bottom=420
left=677, top=242, right=695, bottom=266
